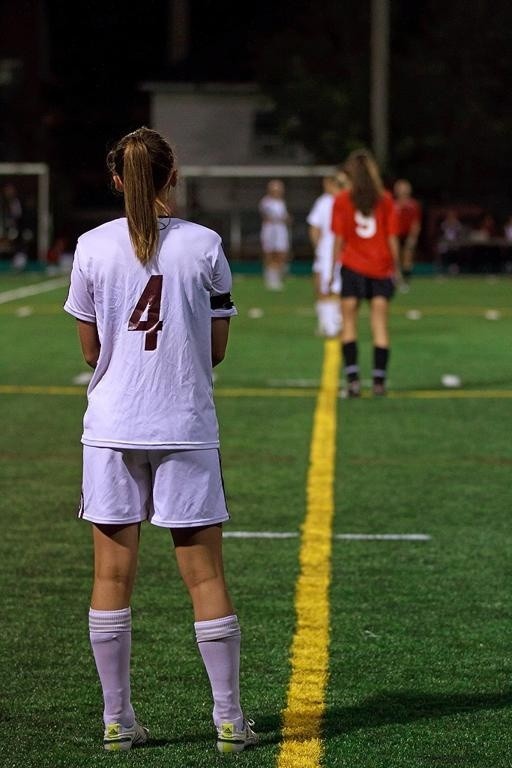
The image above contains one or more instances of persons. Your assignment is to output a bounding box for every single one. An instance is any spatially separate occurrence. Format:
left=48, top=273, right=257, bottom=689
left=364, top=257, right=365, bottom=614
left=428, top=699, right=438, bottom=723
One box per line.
left=258, top=178, right=291, bottom=290
left=306, top=168, right=422, bottom=340
left=325, top=146, right=403, bottom=402
left=59, top=127, right=266, bottom=755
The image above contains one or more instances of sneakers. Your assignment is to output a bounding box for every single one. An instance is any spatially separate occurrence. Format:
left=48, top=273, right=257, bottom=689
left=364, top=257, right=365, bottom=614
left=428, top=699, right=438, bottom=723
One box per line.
left=103, top=719, right=150, bottom=751
left=216, top=719, right=259, bottom=753
left=337, top=384, right=385, bottom=401
